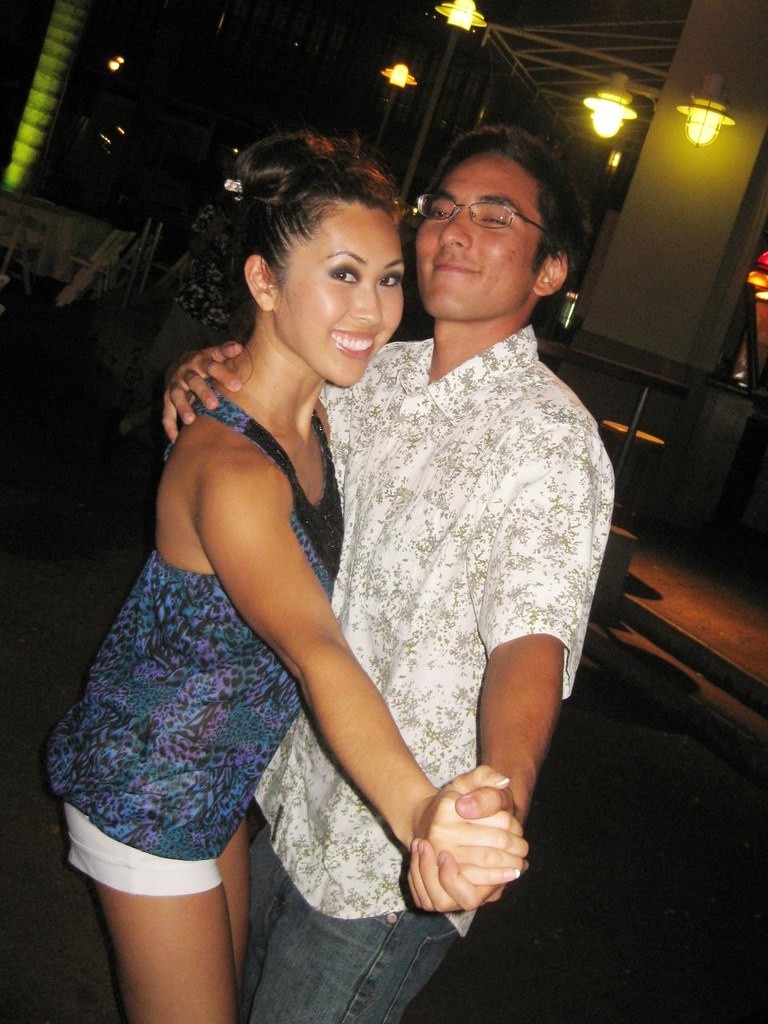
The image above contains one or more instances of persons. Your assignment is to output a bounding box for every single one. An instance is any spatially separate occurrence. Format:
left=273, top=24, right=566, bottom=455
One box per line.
left=47, top=132, right=530, bottom=1024
left=164, top=124, right=614, bottom=1023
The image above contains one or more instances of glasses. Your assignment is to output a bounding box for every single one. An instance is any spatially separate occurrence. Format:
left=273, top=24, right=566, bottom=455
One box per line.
left=416, top=193, right=548, bottom=234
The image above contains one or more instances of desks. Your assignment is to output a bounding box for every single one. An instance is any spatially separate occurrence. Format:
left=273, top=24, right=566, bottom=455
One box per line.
left=534, top=338, right=691, bottom=511
left=2, top=190, right=122, bottom=291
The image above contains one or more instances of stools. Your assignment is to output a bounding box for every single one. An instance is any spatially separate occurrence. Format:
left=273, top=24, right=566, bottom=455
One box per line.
left=599, top=419, right=666, bottom=532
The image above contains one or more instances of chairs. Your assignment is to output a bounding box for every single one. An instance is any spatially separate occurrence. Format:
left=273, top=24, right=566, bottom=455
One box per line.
left=55, top=217, right=171, bottom=309
left=1, top=191, right=30, bottom=294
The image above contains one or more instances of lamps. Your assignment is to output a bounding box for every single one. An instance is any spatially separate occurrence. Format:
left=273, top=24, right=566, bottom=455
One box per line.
left=435, top=0, right=489, bottom=34
left=380, top=60, right=418, bottom=89
left=584, top=70, right=637, bottom=140
left=674, top=74, right=737, bottom=146
